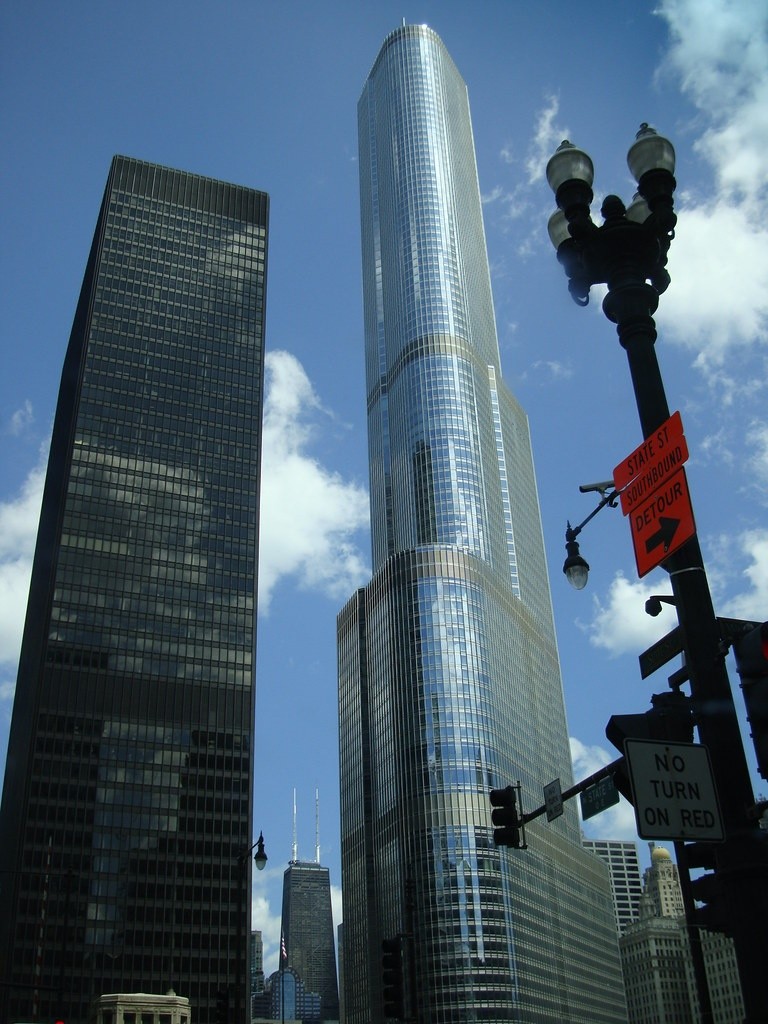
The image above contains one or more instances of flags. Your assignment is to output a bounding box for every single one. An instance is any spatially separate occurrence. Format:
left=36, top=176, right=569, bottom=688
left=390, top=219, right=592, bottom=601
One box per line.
left=282, top=931, right=287, bottom=961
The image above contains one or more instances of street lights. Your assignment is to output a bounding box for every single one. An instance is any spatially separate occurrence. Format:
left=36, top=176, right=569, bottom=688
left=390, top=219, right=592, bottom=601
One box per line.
left=543, top=121, right=768, bottom=1024
left=237, top=831, right=269, bottom=1024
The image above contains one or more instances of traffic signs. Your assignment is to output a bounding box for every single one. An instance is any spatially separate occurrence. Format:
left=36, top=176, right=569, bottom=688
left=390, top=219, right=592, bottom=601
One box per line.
left=629, top=464, right=698, bottom=578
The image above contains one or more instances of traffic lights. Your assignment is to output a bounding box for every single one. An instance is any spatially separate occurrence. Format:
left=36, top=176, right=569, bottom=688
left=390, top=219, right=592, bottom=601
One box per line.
left=489, top=785, right=520, bottom=849
left=681, top=836, right=736, bottom=940
left=606, top=713, right=651, bottom=808
left=381, top=935, right=418, bottom=1022
